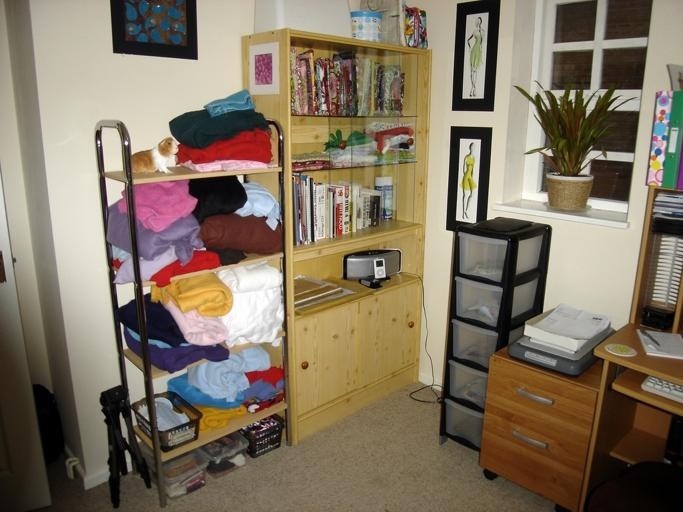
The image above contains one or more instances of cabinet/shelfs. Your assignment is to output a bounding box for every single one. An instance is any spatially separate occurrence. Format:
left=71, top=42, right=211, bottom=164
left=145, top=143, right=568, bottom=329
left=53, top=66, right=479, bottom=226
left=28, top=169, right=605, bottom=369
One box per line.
left=580, top=321, right=683, bottom=511
left=439, top=216, right=552, bottom=449
left=242, top=27, right=425, bottom=448
left=478, top=343, right=601, bottom=512
left=629, top=187, right=683, bottom=335
left=89, top=113, right=287, bottom=506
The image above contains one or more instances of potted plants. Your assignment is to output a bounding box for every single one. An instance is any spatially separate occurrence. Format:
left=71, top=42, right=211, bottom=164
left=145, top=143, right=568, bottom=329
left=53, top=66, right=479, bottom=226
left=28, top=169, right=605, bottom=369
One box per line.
left=513, top=73, right=642, bottom=216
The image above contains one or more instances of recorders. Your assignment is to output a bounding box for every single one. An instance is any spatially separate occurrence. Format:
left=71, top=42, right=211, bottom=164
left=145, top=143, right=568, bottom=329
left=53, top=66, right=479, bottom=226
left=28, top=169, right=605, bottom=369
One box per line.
left=343, top=248, right=401, bottom=289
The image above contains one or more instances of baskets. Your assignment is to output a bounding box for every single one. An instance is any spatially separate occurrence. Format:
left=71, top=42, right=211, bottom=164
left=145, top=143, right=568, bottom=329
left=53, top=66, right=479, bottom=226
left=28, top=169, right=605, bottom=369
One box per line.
left=131, top=391, right=203, bottom=453
left=240, top=414, right=285, bottom=458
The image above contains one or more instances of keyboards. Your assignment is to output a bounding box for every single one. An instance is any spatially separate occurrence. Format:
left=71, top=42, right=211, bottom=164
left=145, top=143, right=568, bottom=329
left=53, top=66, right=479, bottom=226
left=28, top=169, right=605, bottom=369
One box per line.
left=641, top=375, right=683, bottom=404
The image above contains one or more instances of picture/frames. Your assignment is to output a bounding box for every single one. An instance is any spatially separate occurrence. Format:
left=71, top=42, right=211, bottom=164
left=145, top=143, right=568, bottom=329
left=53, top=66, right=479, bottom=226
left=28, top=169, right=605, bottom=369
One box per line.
left=108, top=0, right=199, bottom=61
left=445, top=0, right=505, bottom=239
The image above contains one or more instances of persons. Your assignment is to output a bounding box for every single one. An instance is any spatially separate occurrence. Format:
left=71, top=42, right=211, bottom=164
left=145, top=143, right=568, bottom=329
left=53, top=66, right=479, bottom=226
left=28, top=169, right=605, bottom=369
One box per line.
left=461, top=143, right=475, bottom=220
left=467, top=16, right=485, bottom=98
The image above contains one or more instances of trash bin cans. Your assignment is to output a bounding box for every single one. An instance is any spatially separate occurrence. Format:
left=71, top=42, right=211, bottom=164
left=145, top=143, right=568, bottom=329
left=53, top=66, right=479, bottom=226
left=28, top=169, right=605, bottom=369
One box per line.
left=32, top=384, right=62, bottom=466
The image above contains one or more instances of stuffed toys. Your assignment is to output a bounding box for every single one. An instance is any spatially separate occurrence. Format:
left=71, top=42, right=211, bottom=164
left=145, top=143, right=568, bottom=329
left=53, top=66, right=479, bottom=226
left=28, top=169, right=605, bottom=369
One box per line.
left=132, top=137, right=180, bottom=174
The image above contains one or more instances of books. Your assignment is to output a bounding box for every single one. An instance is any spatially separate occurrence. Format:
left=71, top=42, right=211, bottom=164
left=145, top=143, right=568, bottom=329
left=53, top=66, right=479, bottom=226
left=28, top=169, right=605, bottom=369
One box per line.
left=292, top=172, right=385, bottom=247
left=635, top=328, right=683, bottom=360
left=524, top=308, right=588, bottom=352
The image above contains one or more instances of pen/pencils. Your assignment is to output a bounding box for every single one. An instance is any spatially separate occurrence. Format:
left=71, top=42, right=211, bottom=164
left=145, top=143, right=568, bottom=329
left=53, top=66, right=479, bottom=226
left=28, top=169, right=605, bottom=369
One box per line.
left=639, top=328, right=660, bottom=346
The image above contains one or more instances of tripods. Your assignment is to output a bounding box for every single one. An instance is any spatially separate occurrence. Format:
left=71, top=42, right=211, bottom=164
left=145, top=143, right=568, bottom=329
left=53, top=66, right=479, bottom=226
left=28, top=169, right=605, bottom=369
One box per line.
left=100, top=385, right=151, bottom=509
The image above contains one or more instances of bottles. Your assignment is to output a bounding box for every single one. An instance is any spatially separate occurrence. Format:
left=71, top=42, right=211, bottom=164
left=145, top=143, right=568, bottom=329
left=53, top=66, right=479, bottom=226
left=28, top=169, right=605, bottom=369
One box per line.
left=374, top=176, right=393, bottom=221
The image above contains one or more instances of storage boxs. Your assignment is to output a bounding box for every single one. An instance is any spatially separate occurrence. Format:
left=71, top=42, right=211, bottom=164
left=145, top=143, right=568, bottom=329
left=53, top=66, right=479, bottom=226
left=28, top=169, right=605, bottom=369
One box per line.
left=138, top=430, right=251, bottom=500
